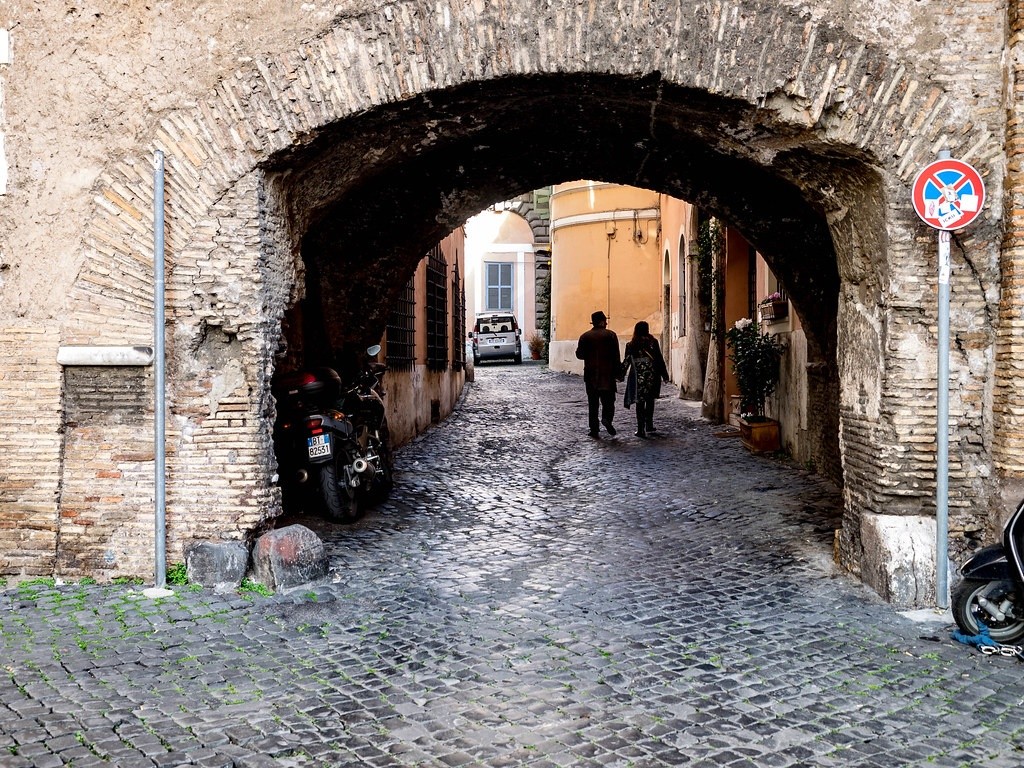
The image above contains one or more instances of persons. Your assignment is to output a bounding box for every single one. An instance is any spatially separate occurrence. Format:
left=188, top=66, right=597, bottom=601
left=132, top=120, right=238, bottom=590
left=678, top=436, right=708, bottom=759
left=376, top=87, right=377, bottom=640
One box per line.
left=575, top=310, right=624, bottom=438
left=617, top=321, right=671, bottom=438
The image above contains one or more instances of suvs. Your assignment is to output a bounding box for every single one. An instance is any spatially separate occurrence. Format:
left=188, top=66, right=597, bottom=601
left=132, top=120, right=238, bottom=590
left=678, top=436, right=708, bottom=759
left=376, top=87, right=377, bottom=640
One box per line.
left=468, top=312, right=522, bottom=365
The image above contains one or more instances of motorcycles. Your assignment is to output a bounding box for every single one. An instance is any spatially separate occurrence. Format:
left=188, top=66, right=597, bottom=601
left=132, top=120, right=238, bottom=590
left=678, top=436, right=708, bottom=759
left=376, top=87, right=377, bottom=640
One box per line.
left=952, top=495, right=1024, bottom=648
left=286, top=344, right=395, bottom=525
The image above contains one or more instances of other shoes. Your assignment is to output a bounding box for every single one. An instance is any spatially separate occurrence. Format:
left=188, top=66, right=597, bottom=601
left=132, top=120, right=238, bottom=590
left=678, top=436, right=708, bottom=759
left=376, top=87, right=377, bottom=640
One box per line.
left=588, top=430, right=599, bottom=438
left=646, top=427, right=657, bottom=432
left=635, top=431, right=645, bottom=437
left=601, top=419, right=616, bottom=435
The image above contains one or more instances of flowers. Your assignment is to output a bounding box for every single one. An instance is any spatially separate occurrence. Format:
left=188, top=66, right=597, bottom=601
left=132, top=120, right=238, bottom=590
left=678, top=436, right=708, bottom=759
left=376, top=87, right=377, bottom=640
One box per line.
left=761, top=292, right=782, bottom=302
left=707, top=312, right=790, bottom=421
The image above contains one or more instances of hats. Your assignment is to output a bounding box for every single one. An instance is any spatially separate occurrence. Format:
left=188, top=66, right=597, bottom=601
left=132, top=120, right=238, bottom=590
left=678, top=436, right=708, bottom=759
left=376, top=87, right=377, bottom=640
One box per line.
left=590, top=311, right=607, bottom=324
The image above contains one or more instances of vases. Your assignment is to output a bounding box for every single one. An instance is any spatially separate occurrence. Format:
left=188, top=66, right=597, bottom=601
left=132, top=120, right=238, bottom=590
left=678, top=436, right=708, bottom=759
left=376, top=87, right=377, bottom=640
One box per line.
left=759, top=301, right=788, bottom=320
left=737, top=417, right=789, bottom=454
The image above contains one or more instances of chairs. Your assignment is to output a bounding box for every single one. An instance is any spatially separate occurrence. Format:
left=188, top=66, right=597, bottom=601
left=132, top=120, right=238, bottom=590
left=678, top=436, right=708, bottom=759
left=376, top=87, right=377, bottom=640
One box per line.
left=501, top=325, right=508, bottom=331
left=483, top=326, right=489, bottom=332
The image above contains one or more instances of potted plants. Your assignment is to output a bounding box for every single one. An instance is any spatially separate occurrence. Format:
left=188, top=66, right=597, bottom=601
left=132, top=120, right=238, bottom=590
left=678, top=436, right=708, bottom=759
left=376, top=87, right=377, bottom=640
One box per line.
left=528, top=333, right=546, bottom=360
